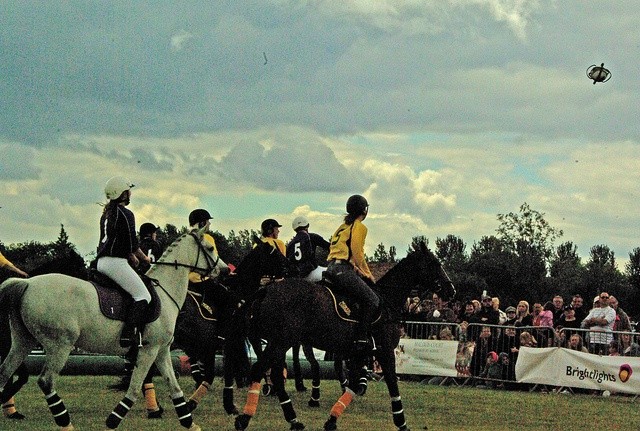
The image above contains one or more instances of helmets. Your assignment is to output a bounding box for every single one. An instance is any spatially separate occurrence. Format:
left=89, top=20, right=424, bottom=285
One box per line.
left=347, top=195, right=370, bottom=212
left=262, top=219, right=282, bottom=229
left=105, top=176, right=135, bottom=200
left=292, top=216, right=309, bottom=230
left=140, top=223, right=159, bottom=234
left=189, top=209, right=213, bottom=222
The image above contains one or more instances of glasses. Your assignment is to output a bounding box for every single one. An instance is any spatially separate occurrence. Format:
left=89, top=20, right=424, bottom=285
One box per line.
left=600, top=296, right=610, bottom=299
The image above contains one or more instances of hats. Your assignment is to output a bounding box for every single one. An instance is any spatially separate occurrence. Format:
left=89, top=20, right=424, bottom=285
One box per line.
left=505, top=306, right=516, bottom=313
left=484, top=296, right=492, bottom=300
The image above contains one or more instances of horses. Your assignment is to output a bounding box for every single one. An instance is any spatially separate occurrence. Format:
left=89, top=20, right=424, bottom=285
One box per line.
left=233, top=242, right=457, bottom=431
left=108, top=236, right=300, bottom=415
left=0, top=223, right=230, bottom=431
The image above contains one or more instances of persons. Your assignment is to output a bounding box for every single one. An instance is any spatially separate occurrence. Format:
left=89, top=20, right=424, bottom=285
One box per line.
left=491, top=297, right=507, bottom=325
left=461, top=301, right=475, bottom=323
left=452, top=300, right=462, bottom=322
left=520, top=331, right=538, bottom=347
left=538, top=301, right=554, bottom=337
left=607, top=340, right=621, bottom=356
left=189, top=209, right=237, bottom=342
left=418, top=300, right=433, bottom=339
left=286, top=217, right=331, bottom=283
left=403, top=297, right=410, bottom=312
left=0, top=251, right=30, bottom=278
left=326, top=195, right=384, bottom=354
left=440, top=329, right=452, bottom=340
left=503, top=306, right=517, bottom=325
left=569, top=334, right=589, bottom=353
left=426, top=295, right=455, bottom=340
left=531, top=303, right=543, bottom=326
left=96, top=175, right=152, bottom=348
left=470, top=299, right=481, bottom=314
left=433, top=293, right=439, bottom=309
left=441, top=324, right=455, bottom=340
left=552, top=295, right=565, bottom=322
left=548, top=325, right=566, bottom=347
left=136, top=223, right=163, bottom=273
left=618, top=329, right=640, bottom=356
left=503, top=301, right=532, bottom=325
left=608, top=295, right=632, bottom=332
left=476, top=326, right=494, bottom=352
left=589, top=296, right=601, bottom=314
left=252, top=219, right=286, bottom=285
left=560, top=294, right=586, bottom=321
left=499, top=325, right=519, bottom=353
left=631, top=315, right=640, bottom=330
left=494, top=351, right=516, bottom=389
left=460, top=320, right=469, bottom=340
left=475, top=351, right=502, bottom=389
left=585, top=292, right=616, bottom=354
left=403, top=303, right=423, bottom=339
left=469, top=295, right=498, bottom=325
left=561, top=307, right=580, bottom=328
left=412, top=297, right=423, bottom=312
left=397, top=324, right=410, bottom=339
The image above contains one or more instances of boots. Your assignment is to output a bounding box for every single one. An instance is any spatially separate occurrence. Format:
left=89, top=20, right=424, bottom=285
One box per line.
left=120, top=300, right=149, bottom=347
